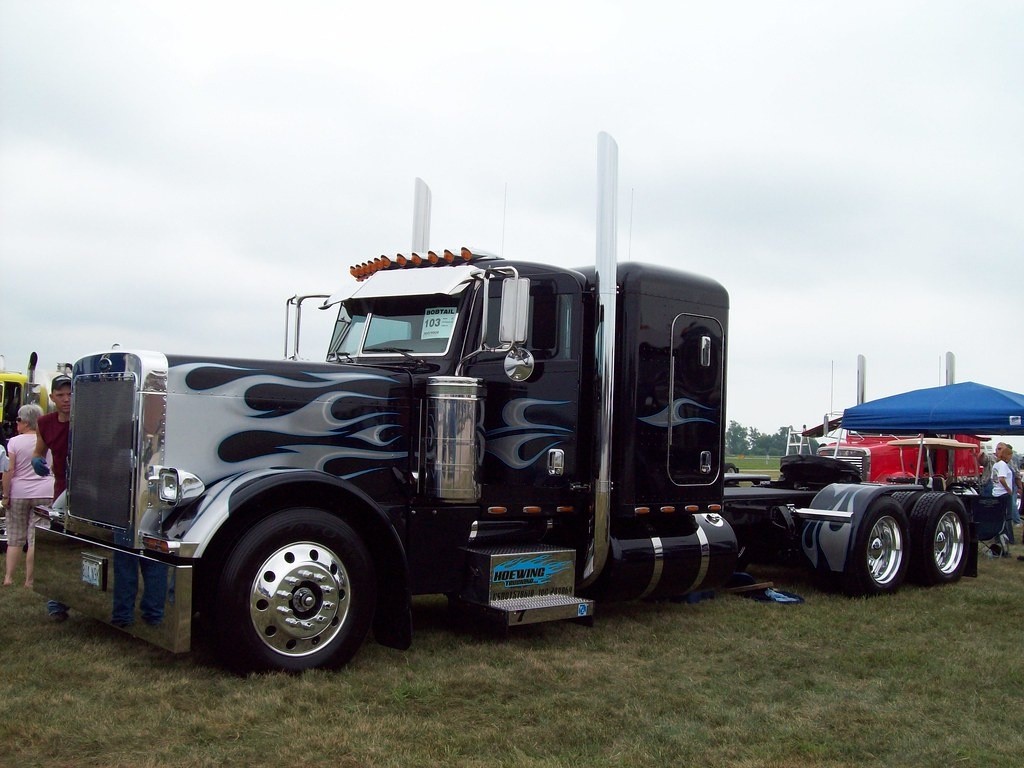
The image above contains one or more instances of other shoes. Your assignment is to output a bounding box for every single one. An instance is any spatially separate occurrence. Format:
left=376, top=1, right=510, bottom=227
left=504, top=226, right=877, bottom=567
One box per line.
left=1016, top=523, right=1021, bottom=527
left=49, top=613, right=67, bottom=622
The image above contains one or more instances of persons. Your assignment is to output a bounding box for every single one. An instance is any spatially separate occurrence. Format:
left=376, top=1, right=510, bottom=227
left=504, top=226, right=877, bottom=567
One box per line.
left=111, top=552, right=168, bottom=627
left=1, top=406, right=55, bottom=588
left=988, top=442, right=1024, bottom=559
left=32, top=376, right=73, bottom=619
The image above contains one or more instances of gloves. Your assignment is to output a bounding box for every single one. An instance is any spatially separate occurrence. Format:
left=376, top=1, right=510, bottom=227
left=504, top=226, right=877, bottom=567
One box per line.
left=32, top=457, right=50, bottom=478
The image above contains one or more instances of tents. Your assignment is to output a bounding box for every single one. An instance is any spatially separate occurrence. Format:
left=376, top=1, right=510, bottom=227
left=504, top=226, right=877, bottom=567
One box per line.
left=832, top=382, right=1024, bottom=486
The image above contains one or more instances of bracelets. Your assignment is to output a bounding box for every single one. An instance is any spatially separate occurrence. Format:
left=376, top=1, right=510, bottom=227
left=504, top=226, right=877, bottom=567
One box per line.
left=2, top=494, right=9, bottom=498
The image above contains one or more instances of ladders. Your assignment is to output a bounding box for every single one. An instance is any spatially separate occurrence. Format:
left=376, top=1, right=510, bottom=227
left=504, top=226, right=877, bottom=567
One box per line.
left=786, top=425, right=812, bottom=456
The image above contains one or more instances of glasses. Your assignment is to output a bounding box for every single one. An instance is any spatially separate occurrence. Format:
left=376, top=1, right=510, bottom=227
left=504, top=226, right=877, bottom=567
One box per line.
left=17, top=417, right=21, bottom=422
left=997, top=447, right=1001, bottom=450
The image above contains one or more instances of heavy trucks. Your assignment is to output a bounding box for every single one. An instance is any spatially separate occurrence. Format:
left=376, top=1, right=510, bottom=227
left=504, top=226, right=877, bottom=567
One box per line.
left=29, top=128, right=1008, bottom=676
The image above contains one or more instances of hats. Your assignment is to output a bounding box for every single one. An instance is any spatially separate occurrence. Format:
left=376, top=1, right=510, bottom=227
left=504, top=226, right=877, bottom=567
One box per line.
left=52, top=375, right=72, bottom=391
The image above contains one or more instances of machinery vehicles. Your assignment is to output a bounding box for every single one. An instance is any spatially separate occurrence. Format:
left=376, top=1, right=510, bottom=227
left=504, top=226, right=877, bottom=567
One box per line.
left=0, top=352, right=50, bottom=447
left=799, top=352, right=994, bottom=490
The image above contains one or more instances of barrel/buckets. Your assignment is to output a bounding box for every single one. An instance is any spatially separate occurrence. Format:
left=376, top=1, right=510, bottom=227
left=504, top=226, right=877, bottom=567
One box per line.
left=423, top=377, right=481, bottom=503
